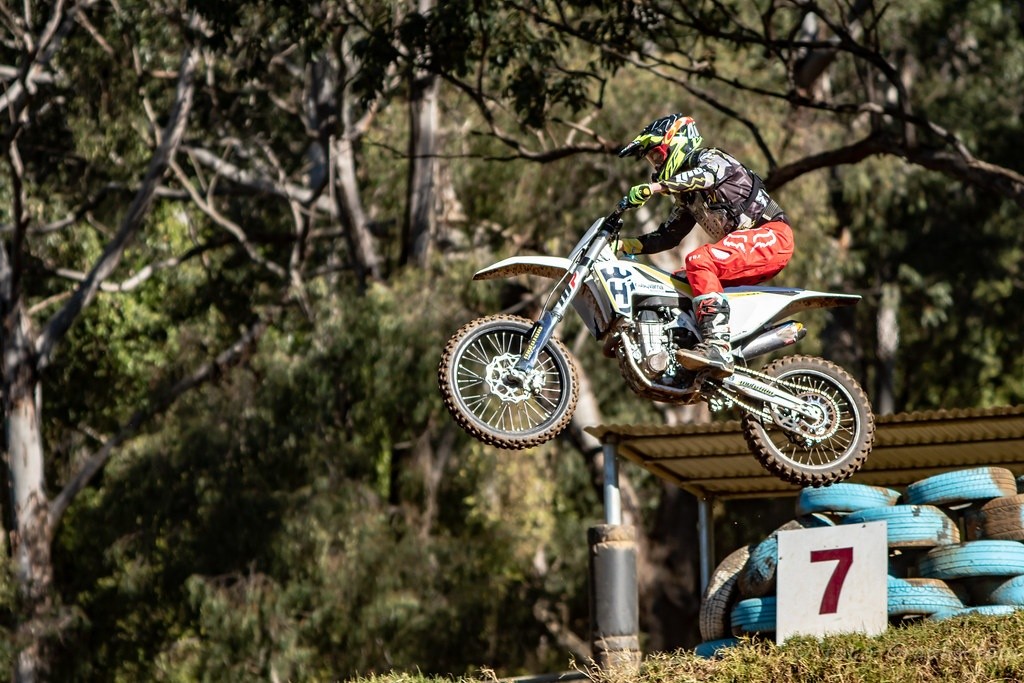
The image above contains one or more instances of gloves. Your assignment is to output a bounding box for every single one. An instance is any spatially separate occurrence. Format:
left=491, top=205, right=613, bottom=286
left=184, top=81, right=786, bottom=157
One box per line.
left=609, top=236, right=642, bottom=256
left=629, top=182, right=654, bottom=209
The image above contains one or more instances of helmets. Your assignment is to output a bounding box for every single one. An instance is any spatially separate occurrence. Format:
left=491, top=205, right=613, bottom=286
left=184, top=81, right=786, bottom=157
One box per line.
left=617, top=112, right=705, bottom=183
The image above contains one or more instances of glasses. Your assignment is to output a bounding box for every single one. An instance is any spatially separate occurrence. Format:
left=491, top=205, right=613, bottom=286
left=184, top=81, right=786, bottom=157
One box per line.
left=644, top=146, right=667, bottom=169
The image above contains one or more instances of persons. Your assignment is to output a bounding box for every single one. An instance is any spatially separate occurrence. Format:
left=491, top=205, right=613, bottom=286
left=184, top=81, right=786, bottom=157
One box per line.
left=611, top=112, right=794, bottom=376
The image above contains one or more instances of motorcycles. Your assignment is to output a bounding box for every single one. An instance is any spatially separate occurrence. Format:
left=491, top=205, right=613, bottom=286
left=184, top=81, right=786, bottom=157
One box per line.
left=438, top=185, right=876, bottom=488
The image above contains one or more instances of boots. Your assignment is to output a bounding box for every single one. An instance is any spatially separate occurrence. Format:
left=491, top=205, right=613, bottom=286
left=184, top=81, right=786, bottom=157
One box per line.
left=675, top=292, right=734, bottom=379
